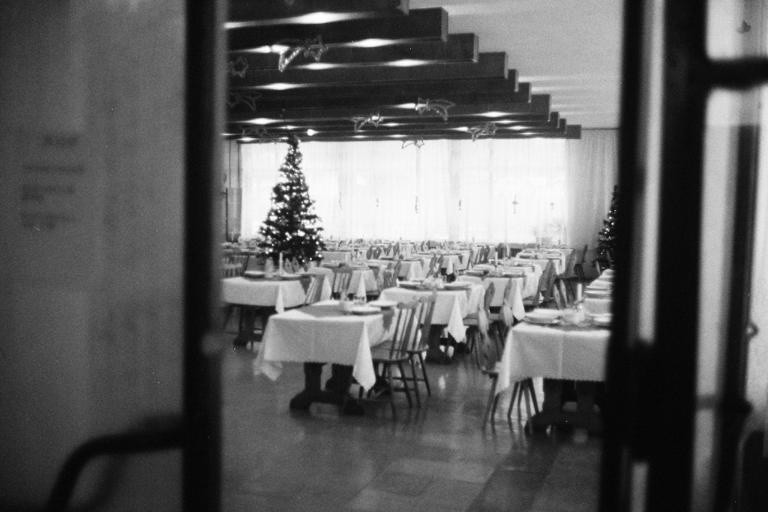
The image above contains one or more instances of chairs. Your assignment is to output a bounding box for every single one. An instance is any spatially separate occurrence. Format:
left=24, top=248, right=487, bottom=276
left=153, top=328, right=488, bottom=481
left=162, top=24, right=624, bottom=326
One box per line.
left=218, top=235, right=615, bottom=450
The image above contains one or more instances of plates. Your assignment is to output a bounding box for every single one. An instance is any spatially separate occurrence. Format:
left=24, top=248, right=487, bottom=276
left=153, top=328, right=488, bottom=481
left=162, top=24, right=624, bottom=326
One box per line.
left=244, top=270, right=302, bottom=279
left=521, top=306, right=611, bottom=327
left=399, top=278, right=473, bottom=289
left=584, top=284, right=611, bottom=298
left=466, top=267, right=528, bottom=276
left=311, top=300, right=399, bottom=314
